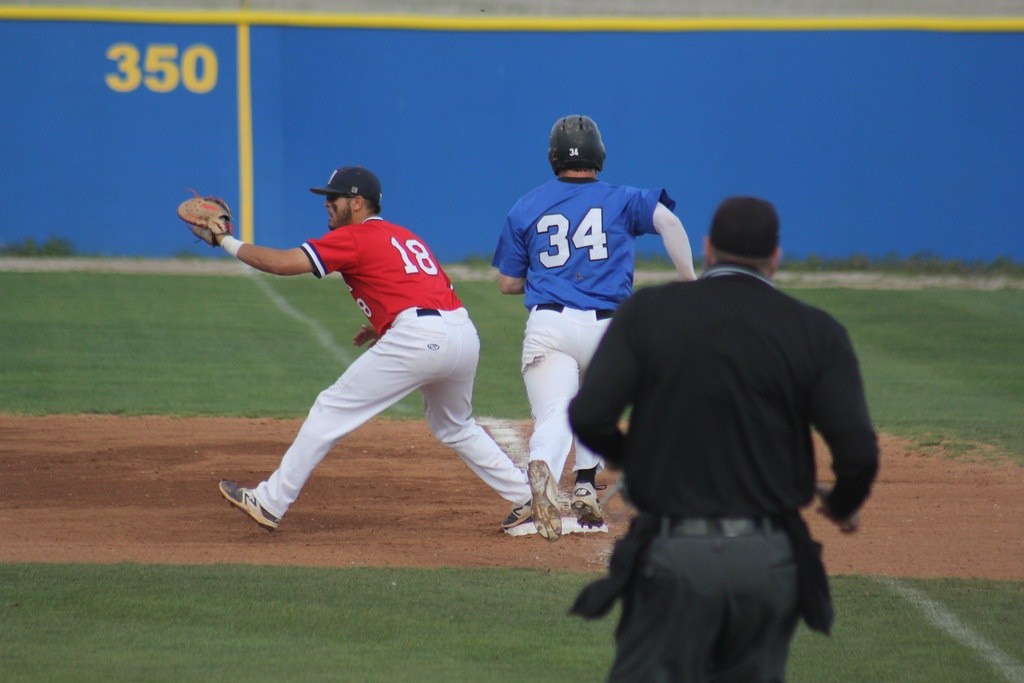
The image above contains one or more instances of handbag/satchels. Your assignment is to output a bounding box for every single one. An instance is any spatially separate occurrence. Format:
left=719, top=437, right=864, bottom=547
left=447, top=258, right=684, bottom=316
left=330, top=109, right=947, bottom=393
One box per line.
left=779, top=511, right=834, bottom=637
left=567, top=513, right=661, bottom=620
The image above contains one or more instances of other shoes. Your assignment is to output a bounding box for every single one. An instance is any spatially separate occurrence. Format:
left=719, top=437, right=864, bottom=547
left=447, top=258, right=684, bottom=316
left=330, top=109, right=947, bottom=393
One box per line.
left=527, top=461, right=562, bottom=543
left=571, top=482, right=607, bottom=528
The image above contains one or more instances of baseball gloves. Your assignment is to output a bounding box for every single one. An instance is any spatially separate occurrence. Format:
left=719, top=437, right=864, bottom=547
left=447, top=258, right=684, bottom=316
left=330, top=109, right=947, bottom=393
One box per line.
left=175, top=193, right=238, bottom=250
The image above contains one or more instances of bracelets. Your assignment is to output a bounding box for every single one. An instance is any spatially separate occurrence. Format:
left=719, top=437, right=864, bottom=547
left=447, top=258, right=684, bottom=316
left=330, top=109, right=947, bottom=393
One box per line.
left=220, top=235, right=246, bottom=259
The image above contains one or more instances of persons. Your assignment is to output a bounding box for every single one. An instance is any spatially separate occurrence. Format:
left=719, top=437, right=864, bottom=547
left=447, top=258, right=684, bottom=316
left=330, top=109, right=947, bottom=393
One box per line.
left=178, top=166, right=534, bottom=530
left=492, top=115, right=697, bottom=542
left=568, top=196, right=881, bottom=683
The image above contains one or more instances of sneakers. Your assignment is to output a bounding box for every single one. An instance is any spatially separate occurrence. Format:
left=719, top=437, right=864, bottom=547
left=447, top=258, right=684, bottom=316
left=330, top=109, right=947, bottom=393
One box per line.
left=501, top=499, right=531, bottom=528
left=219, top=480, right=280, bottom=530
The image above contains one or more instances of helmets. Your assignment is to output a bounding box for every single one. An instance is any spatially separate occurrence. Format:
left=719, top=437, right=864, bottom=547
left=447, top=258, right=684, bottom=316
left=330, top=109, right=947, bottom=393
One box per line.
left=548, top=116, right=606, bottom=176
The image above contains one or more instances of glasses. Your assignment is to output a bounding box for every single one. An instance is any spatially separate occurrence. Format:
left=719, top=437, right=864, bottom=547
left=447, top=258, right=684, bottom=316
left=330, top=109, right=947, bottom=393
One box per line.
left=327, top=194, right=357, bottom=202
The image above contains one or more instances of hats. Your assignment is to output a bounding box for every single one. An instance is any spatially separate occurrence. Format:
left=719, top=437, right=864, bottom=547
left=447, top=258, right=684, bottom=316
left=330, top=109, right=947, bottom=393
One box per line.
left=709, top=196, right=780, bottom=257
left=309, top=165, right=381, bottom=201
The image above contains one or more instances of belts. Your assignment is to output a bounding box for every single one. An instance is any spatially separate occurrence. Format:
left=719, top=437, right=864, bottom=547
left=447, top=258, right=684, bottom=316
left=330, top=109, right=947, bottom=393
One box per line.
left=535, top=303, right=614, bottom=320
left=417, top=307, right=441, bottom=316
left=670, top=517, right=756, bottom=537
